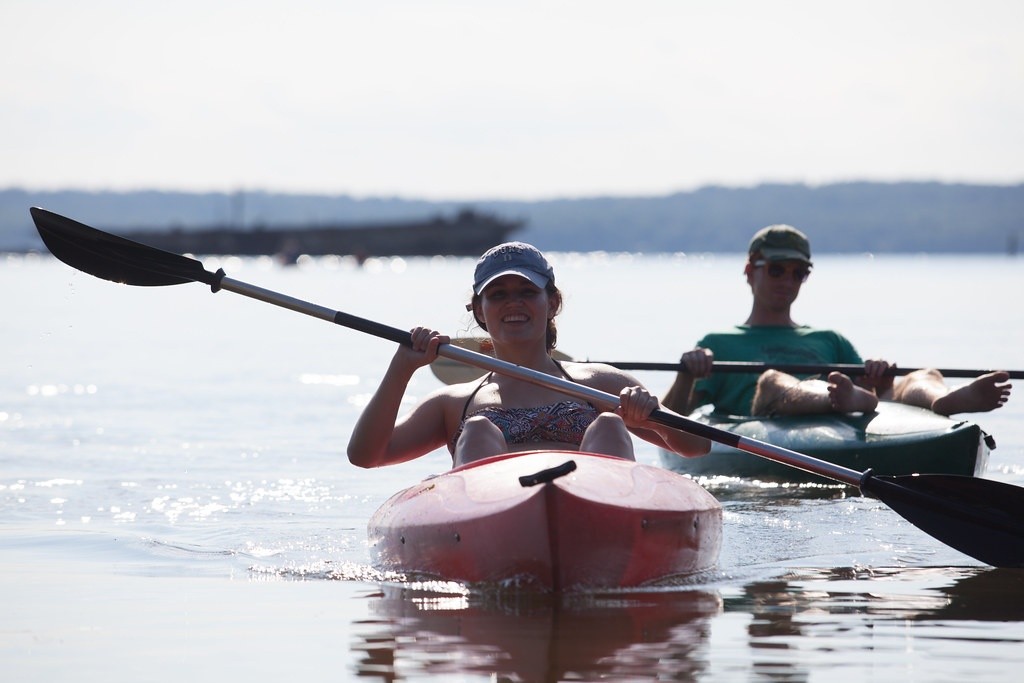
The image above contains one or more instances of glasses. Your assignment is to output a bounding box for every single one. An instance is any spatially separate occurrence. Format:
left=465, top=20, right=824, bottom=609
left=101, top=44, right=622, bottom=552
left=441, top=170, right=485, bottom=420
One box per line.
left=752, top=258, right=810, bottom=282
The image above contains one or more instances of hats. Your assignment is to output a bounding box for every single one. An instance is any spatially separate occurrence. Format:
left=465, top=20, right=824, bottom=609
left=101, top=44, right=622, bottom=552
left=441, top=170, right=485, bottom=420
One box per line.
left=465, top=241, right=555, bottom=331
left=749, top=224, right=812, bottom=270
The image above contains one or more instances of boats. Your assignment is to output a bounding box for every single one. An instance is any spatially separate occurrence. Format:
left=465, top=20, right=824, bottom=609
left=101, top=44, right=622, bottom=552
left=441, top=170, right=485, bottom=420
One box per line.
left=659, top=400, right=980, bottom=485
left=367, top=449, right=723, bottom=591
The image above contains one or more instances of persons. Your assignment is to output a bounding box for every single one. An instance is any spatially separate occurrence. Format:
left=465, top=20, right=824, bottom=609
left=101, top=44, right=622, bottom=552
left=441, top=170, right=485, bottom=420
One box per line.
left=347, top=242, right=712, bottom=470
left=662, top=225, right=1012, bottom=418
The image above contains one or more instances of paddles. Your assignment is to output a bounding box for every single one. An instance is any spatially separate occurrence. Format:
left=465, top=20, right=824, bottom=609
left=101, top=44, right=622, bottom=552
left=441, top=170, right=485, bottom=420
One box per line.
left=29, top=207, right=1024, bottom=569
left=428, top=334, right=1024, bottom=386
